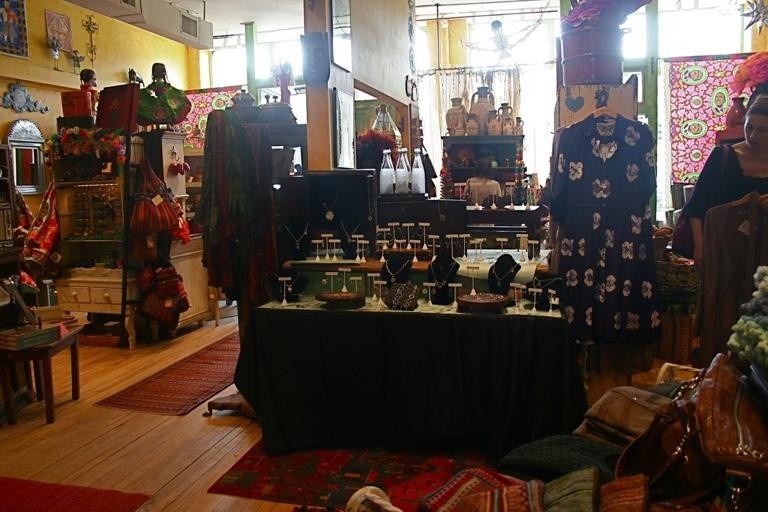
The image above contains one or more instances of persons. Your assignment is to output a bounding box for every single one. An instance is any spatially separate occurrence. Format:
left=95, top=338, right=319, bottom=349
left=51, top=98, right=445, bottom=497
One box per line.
left=683, top=96, right=768, bottom=274
left=80, top=69, right=98, bottom=114
left=0, top=0, right=19, bottom=51
left=464, top=161, right=502, bottom=226
left=137, top=63, right=191, bottom=131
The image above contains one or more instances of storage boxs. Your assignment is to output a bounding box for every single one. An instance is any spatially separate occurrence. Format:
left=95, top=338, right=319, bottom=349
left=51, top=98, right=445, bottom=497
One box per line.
left=61, top=90, right=91, bottom=118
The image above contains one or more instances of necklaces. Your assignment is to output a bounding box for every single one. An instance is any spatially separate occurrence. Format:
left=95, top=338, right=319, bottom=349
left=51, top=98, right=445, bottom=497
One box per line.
left=492, top=261, right=519, bottom=286
left=285, top=220, right=310, bottom=250
left=341, top=220, right=363, bottom=242
left=431, top=261, right=456, bottom=288
left=274, top=271, right=300, bottom=291
left=385, top=259, right=410, bottom=283
left=533, top=276, right=563, bottom=296
left=320, top=191, right=339, bottom=220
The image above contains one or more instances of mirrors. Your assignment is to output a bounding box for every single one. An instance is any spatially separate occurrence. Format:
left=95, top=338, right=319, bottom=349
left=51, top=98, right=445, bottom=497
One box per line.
left=4, top=119, right=46, bottom=196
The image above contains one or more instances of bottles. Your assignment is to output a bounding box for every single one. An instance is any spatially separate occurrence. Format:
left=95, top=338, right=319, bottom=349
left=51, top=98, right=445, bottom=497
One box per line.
left=384, top=149, right=395, bottom=193
left=455, top=129, right=466, bottom=136
left=400, top=147, right=412, bottom=191
left=379, top=151, right=393, bottom=194
left=395, top=149, right=408, bottom=191
left=412, top=148, right=425, bottom=194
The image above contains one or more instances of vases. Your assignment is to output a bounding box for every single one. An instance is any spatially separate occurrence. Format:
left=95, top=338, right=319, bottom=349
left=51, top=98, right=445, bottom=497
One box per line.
left=445, top=86, right=524, bottom=136
left=726, top=98, right=747, bottom=127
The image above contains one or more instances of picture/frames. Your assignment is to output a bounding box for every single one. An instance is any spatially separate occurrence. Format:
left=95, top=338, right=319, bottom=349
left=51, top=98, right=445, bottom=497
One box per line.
left=45, top=9, right=73, bottom=52
left=0, top=0, right=30, bottom=61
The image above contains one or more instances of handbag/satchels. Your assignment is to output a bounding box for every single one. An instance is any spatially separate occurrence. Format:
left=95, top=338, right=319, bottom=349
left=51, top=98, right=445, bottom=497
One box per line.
left=156, top=246, right=170, bottom=267
left=736, top=362, right=767, bottom=413
left=543, top=469, right=596, bottom=510
left=696, top=354, right=766, bottom=465
left=617, top=380, right=695, bottom=498
left=130, top=163, right=179, bottom=230
left=586, top=386, right=672, bottom=447
left=143, top=294, right=175, bottom=326
left=422, top=468, right=543, bottom=512
left=126, top=253, right=145, bottom=271
left=155, top=268, right=179, bottom=297
left=500, top=433, right=617, bottom=480
left=177, top=281, right=190, bottom=314
left=135, top=235, right=158, bottom=260
left=597, top=474, right=646, bottom=512
left=672, top=199, right=694, bottom=258
left=137, top=267, right=153, bottom=291
left=650, top=486, right=722, bottom=511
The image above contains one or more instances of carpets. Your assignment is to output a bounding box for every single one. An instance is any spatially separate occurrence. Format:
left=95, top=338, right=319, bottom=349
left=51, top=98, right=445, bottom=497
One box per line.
left=0, top=476, right=151, bottom=512
left=207, top=436, right=489, bottom=512
left=93, top=330, right=241, bottom=416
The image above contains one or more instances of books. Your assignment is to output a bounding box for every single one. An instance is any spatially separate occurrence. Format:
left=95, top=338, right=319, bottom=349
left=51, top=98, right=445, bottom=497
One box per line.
left=0, top=323, right=60, bottom=352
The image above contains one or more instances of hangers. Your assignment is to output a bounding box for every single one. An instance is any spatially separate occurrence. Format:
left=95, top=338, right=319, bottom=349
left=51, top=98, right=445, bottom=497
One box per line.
left=591, top=90, right=618, bottom=143
left=729, top=190, right=768, bottom=209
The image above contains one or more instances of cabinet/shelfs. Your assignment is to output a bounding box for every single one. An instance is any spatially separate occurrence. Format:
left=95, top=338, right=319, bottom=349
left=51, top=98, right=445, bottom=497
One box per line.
left=441, top=134, right=526, bottom=199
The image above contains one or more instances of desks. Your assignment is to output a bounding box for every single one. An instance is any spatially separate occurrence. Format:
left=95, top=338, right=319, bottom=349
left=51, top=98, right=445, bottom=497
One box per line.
left=234, top=292, right=589, bottom=455
left=0, top=324, right=83, bottom=424
left=53, top=135, right=158, bottom=350
